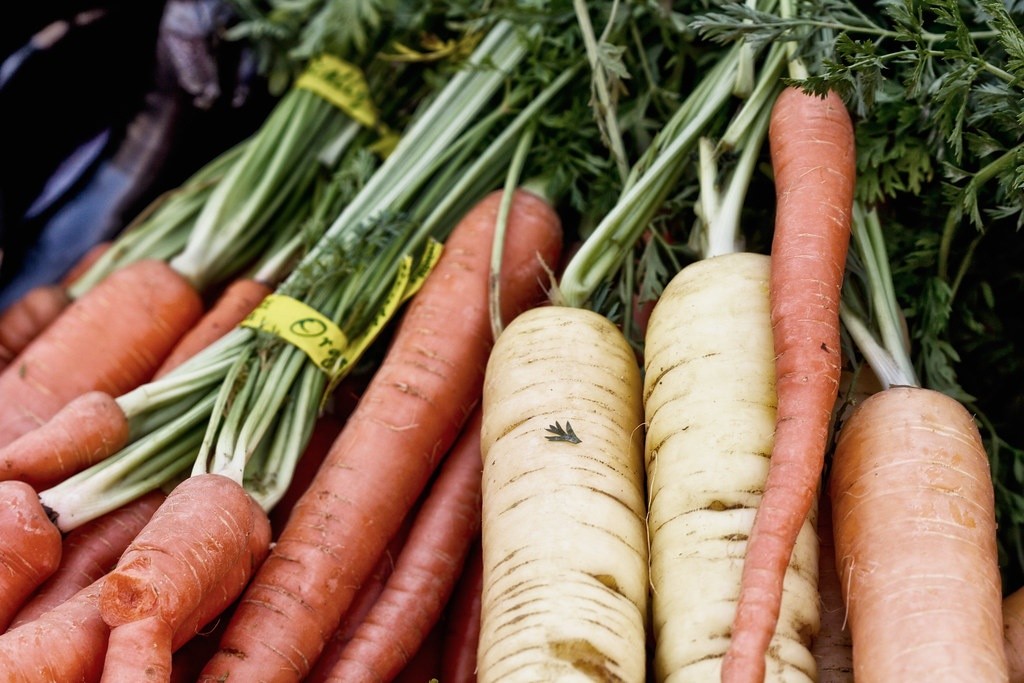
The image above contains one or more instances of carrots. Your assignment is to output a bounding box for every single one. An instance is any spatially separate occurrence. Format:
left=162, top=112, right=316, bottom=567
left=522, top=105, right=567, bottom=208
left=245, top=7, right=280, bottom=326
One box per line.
left=0, top=79, right=1024, bottom=683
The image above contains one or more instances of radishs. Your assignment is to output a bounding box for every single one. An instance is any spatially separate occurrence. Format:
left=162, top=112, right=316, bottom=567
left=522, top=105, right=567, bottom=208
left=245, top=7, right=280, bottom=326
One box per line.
left=642, top=250, right=819, bottom=683
left=477, top=304, right=649, bottom=683
